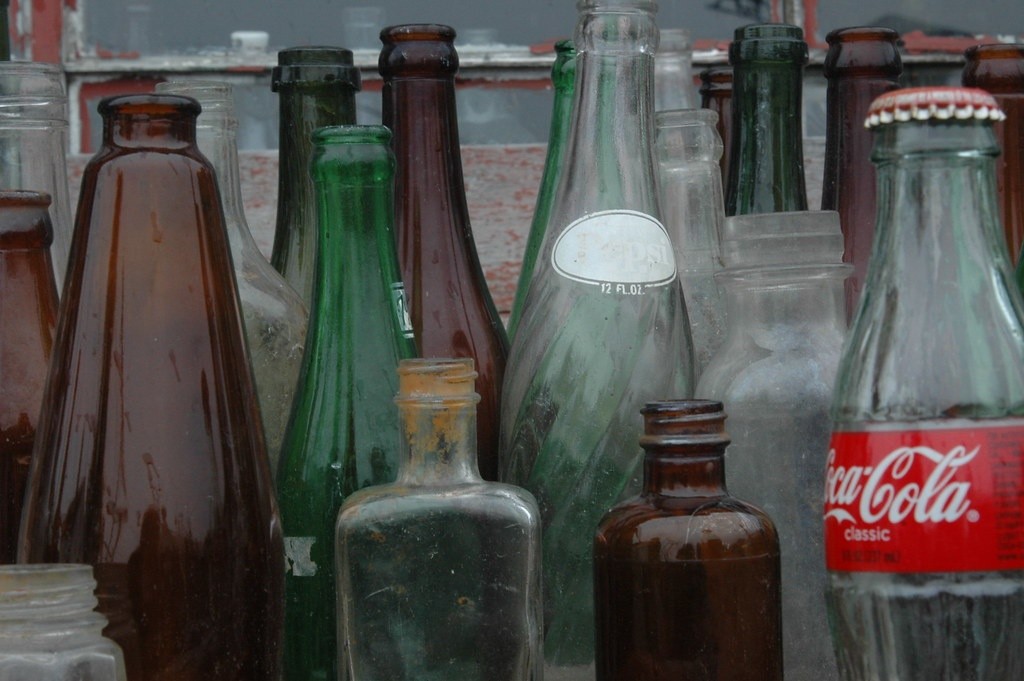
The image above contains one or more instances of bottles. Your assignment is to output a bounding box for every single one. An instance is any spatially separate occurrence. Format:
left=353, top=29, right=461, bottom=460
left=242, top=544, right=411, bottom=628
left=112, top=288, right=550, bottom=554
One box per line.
left=0, top=0, right=1024, bottom=681
